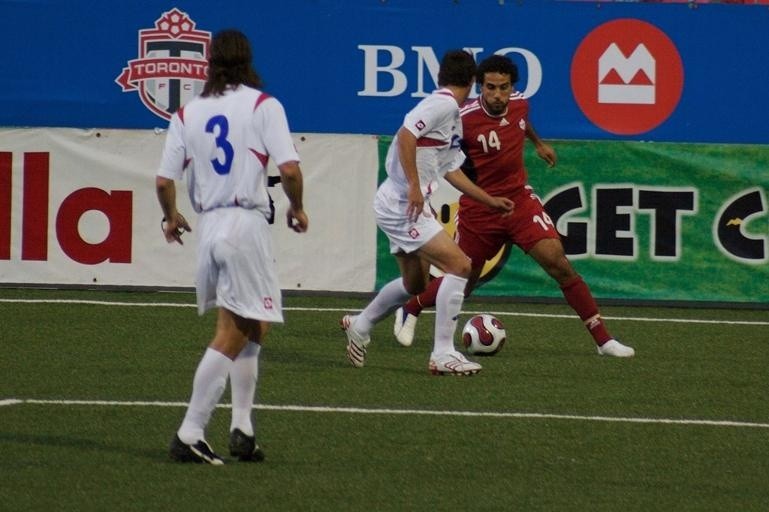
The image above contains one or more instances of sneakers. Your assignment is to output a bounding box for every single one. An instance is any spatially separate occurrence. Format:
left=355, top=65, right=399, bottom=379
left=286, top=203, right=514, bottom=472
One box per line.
left=339, top=314, right=371, bottom=368
left=429, top=350, right=482, bottom=377
left=169, top=433, right=226, bottom=467
left=393, top=306, right=419, bottom=346
left=230, top=428, right=266, bottom=462
left=597, top=339, right=635, bottom=359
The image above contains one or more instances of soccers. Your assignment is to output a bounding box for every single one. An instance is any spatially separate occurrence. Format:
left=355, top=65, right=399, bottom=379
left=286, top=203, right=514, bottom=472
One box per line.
left=461, top=314, right=506, bottom=356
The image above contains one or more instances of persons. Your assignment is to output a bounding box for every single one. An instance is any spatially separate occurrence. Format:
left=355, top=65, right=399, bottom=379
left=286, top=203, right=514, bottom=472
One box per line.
left=339, top=50, right=515, bottom=377
left=156, top=29, right=308, bottom=465
left=394, top=54, right=635, bottom=358
